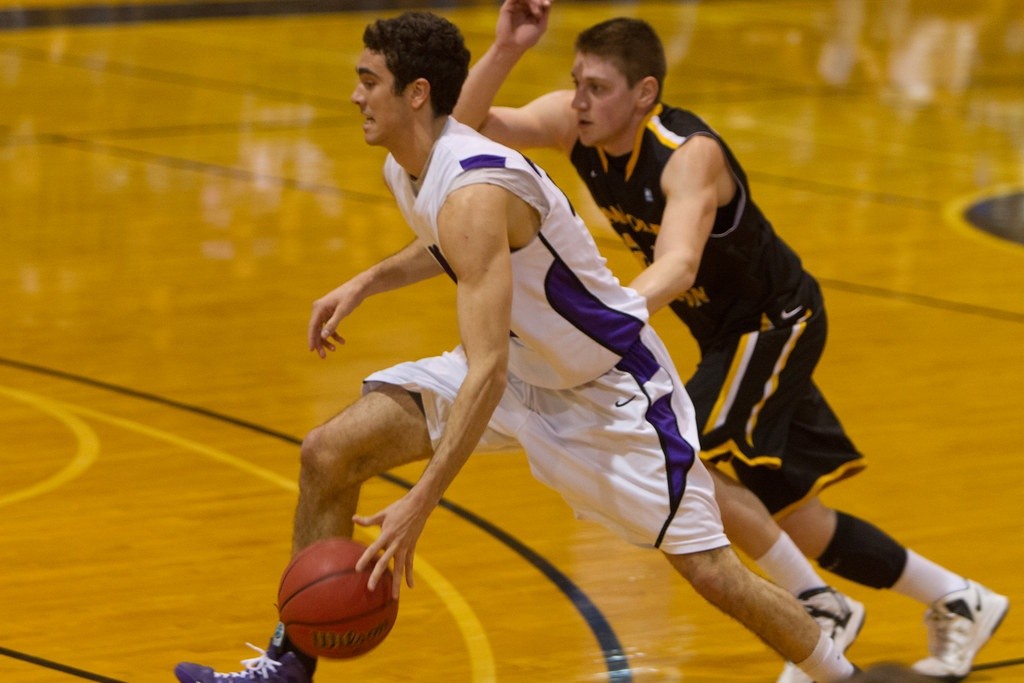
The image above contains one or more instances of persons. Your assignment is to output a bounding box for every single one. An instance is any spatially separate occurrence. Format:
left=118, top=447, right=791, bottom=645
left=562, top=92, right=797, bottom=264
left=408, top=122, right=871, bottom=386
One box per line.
left=452, top=0, right=1007, bottom=683
left=173, top=11, right=864, bottom=683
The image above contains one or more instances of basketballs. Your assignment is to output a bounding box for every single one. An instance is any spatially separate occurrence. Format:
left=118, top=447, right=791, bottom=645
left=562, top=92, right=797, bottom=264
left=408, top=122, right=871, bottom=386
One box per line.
left=278, top=539, right=399, bottom=659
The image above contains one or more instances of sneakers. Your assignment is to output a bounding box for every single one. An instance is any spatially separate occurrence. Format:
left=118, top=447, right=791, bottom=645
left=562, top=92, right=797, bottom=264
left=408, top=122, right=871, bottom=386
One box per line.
left=174, top=621, right=311, bottom=683
left=776, top=586, right=865, bottom=683
left=911, top=577, right=1009, bottom=677
left=809, top=661, right=867, bottom=683
